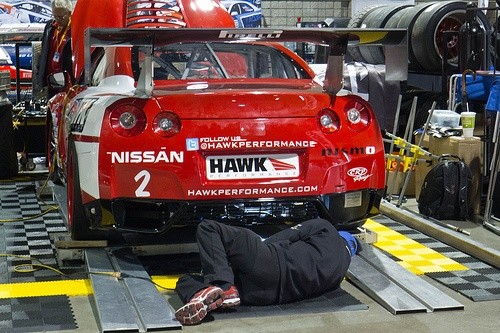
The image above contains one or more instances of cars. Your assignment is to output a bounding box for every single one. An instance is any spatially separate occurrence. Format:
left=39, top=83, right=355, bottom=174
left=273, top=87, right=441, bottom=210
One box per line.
left=45, top=0, right=407, bottom=240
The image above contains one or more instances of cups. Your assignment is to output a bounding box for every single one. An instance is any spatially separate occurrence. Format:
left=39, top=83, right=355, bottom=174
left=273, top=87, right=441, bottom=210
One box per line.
left=461, top=112, right=476, bottom=137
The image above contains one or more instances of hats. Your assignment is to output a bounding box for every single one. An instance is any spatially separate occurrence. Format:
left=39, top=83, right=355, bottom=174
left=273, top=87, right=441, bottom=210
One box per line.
left=338, top=230, right=359, bottom=256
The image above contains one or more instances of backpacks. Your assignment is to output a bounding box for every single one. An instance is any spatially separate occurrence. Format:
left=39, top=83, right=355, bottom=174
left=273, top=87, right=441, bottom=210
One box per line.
left=418, top=155, right=472, bottom=220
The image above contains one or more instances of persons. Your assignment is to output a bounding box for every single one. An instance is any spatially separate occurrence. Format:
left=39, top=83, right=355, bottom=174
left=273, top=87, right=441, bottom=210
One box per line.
left=37, top=0, right=73, bottom=99
left=175, top=218, right=358, bottom=325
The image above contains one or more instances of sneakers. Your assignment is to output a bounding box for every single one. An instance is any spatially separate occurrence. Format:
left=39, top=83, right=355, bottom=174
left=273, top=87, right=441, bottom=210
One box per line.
left=173, top=285, right=242, bottom=326
